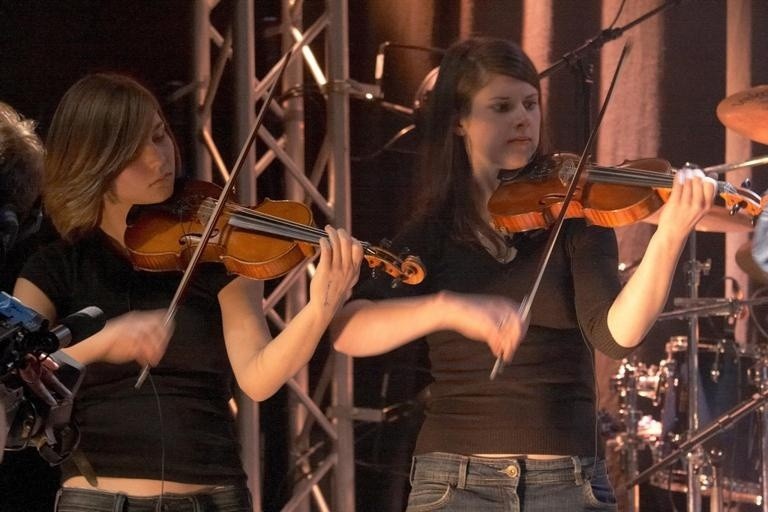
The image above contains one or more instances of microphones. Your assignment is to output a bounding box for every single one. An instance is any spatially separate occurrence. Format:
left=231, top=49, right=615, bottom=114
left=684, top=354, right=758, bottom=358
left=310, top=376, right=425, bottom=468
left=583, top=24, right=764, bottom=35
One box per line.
left=378, top=102, right=414, bottom=121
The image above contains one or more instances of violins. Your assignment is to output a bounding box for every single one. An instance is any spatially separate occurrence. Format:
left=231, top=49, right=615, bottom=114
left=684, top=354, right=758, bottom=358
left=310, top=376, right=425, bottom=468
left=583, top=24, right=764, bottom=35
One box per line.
left=487, top=150, right=762, bottom=233
left=122, top=176, right=427, bottom=290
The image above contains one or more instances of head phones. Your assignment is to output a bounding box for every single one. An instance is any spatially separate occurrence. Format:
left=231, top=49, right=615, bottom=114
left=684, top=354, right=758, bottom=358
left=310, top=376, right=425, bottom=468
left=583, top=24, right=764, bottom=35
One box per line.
left=0, top=211, right=19, bottom=259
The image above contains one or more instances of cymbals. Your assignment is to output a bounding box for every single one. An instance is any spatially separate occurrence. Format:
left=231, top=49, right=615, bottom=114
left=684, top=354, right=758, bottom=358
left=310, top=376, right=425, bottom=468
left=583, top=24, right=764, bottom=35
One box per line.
left=716, top=84, right=768, bottom=145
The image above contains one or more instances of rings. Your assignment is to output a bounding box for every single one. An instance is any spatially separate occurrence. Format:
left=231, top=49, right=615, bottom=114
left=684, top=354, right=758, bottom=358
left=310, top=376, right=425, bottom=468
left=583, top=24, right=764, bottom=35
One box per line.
left=495, top=320, right=503, bottom=330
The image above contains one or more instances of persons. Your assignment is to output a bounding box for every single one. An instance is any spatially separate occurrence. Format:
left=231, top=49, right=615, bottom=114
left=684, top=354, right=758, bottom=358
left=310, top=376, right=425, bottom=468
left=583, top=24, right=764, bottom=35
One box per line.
left=9, top=63, right=364, bottom=511
left=1, top=104, right=49, bottom=293
left=330, top=36, right=720, bottom=512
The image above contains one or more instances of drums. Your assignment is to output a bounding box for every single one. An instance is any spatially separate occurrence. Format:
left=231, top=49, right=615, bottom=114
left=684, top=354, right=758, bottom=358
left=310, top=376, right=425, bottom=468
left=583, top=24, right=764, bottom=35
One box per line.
left=651, top=336, right=767, bottom=507
